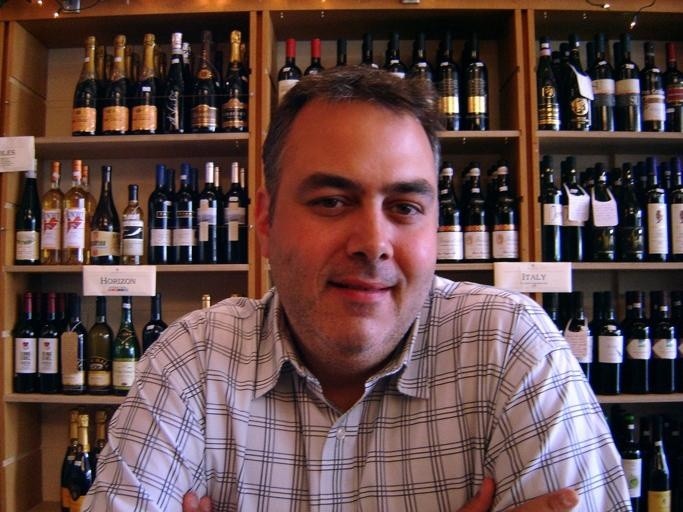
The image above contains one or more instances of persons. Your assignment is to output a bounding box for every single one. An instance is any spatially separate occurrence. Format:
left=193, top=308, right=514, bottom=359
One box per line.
left=77, top=63, right=640, bottom=512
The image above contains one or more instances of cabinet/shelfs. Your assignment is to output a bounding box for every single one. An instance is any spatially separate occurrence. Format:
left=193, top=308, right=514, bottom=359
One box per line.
left=1, top=2, right=258, bottom=512
left=257, top=2, right=529, bottom=301
left=529, top=0, right=681, bottom=511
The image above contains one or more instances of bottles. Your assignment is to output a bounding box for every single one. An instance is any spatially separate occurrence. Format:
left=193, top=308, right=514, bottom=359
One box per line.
left=544, top=288, right=683, bottom=395
left=39, top=159, right=99, bottom=265
left=302, top=36, right=327, bottom=91
left=540, top=157, right=683, bottom=262
left=333, top=38, right=349, bottom=67
left=86, top=294, right=113, bottom=395
left=146, top=158, right=247, bottom=264
left=438, top=163, right=522, bottom=262
left=382, top=32, right=409, bottom=82
left=13, top=291, right=85, bottom=395
left=409, top=28, right=435, bottom=105
left=536, top=29, right=683, bottom=132
left=141, top=292, right=169, bottom=352
left=355, top=28, right=380, bottom=69
left=461, top=29, right=489, bottom=133
left=72, top=28, right=247, bottom=136
left=13, top=159, right=41, bottom=266
left=276, top=37, right=301, bottom=105
left=60, top=409, right=108, bottom=512
left=112, top=292, right=140, bottom=398
left=601, top=412, right=682, bottom=511
left=90, top=164, right=123, bottom=263
left=121, top=184, right=146, bottom=265
left=434, top=31, right=459, bottom=132
left=199, top=294, right=212, bottom=309
